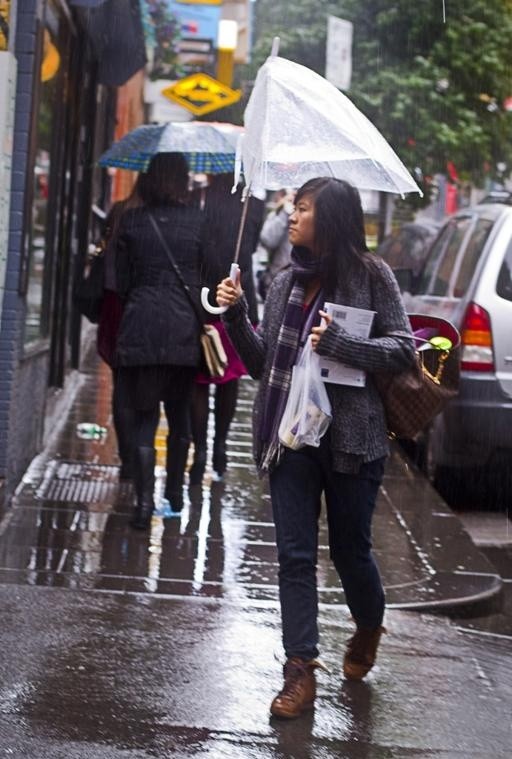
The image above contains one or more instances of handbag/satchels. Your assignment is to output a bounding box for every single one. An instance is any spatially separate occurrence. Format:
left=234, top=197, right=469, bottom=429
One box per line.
left=378, top=348, right=460, bottom=442
left=76, top=256, right=104, bottom=324
left=200, top=322, right=228, bottom=380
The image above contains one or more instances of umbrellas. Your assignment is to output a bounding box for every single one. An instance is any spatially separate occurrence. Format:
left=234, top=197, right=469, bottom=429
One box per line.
left=94, top=120, right=247, bottom=176
left=198, top=35, right=423, bottom=316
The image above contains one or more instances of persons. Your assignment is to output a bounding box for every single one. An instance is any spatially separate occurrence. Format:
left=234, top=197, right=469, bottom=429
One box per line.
left=215, top=175, right=418, bottom=718
left=76, top=203, right=134, bottom=482
left=186, top=177, right=302, bottom=482
left=81, top=519, right=236, bottom=600
left=108, top=152, right=214, bottom=530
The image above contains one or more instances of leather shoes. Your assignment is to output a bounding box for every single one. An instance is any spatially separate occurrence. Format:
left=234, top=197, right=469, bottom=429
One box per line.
left=270, top=660, right=319, bottom=718
left=342, top=622, right=383, bottom=680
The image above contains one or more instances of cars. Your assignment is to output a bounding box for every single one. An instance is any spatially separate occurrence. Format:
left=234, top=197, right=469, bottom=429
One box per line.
left=402, top=202, right=512, bottom=494
left=377, top=223, right=445, bottom=289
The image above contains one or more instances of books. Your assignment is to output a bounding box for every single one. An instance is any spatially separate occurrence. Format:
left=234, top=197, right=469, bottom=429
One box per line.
left=315, top=302, right=376, bottom=388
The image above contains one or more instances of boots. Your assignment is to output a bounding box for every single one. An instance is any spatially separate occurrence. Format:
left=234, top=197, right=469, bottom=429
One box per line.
left=134, top=447, right=155, bottom=531
left=164, top=435, right=187, bottom=510
left=213, top=400, right=237, bottom=475
left=190, top=407, right=210, bottom=483
